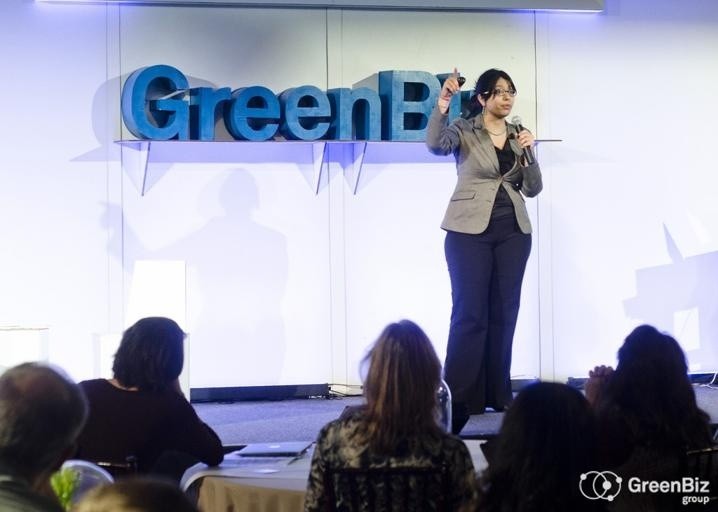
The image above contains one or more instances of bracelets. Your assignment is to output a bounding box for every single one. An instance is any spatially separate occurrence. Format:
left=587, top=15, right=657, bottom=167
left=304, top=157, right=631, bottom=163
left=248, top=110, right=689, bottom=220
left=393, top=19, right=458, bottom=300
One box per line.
left=439, top=93, right=451, bottom=102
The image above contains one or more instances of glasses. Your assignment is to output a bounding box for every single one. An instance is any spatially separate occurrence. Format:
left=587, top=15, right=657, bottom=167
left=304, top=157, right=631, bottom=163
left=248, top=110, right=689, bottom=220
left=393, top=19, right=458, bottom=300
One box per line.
left=483, top=89, right=517, bottom=97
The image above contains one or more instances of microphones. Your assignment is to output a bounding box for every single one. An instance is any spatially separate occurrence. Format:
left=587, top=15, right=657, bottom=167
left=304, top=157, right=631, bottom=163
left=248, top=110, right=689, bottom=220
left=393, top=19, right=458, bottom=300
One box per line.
left=512, top=116, right=535, bottom=166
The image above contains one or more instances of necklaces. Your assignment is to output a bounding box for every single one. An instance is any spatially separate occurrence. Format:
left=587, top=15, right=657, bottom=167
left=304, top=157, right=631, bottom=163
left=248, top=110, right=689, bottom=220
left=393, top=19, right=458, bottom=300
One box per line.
left=488, top=127, right=507, bottom=138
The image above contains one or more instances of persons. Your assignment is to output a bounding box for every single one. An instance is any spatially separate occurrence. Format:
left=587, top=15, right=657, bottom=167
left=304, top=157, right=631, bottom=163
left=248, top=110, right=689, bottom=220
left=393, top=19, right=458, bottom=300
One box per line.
left=584, top=317, right=716, bottom=484
left=0, top=363, right=90, bottom=512
left=68, top=476, right=201, bottom=512
left=303, top=320, right=477, bottom=510
left=467, top=374, right=602, bottom=510
left=70, top=315, right=225, bottom=500
left=425, top=67, right=544, bottom=414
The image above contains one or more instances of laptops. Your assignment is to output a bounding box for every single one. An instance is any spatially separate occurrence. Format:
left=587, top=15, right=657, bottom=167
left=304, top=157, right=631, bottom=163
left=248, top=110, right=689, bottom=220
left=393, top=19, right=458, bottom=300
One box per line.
left=239, top=441, right=312, bottom=455
